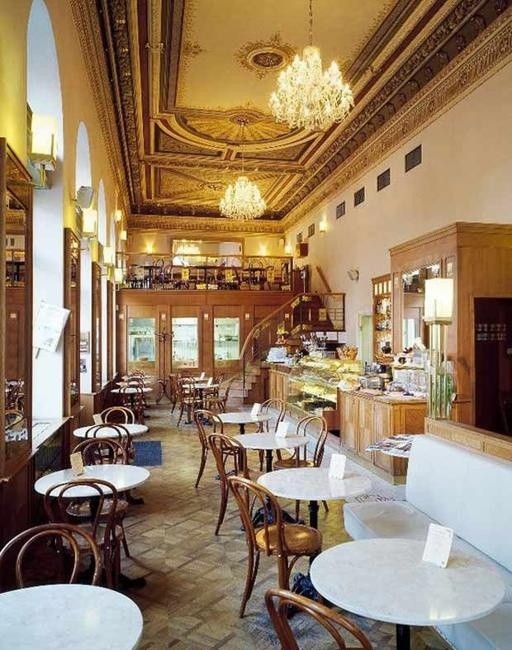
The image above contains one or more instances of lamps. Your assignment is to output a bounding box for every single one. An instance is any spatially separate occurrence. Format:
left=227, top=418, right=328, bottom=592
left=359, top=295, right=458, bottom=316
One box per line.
left=266, top=0, right=355, bottom=134
left=100, top=248, right=125, bottom=291
left=218, top=123, right=267, bottom=223
left=70, top=207, right=99, bottom=251
left=347, top=269, right=359, bottom=283
left=418, top=274, right=456, bottom=418
left=319, top=220, right=327, bottom=234
left=14, top=112, right=60, bottom=193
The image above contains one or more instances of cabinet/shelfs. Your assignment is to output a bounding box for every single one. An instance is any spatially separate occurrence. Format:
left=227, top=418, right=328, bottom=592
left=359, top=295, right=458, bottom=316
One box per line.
left=268, top=369, right=340, bottom=430
left=340, top=391, right=425, bottom=476
left=372, top=272, right=392, bottom=365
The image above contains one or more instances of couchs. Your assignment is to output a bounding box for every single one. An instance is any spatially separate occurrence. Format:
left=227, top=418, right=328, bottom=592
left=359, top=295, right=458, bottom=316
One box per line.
left=343, top=434, right=511, bottom=649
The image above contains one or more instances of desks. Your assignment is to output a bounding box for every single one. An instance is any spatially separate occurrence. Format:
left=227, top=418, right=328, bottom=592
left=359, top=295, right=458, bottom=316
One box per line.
left=34, top=465, right=151, bottom=524
left=222, top=433, right=310, bottom=531
left=309, top=538, right=505, bottom=650
left=212, top=412, right=273, bottom=480
left=73, top=423, right=148, bottom=462
left=0, top=584, right=143, bottom=650
left=257, top=467, right=374, bottom=620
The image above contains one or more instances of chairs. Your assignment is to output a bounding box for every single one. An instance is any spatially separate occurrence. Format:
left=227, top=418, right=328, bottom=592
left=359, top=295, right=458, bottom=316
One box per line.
left=195, top=409, right=238, bottom=488
left=256, top=398, right=286, bottom=472
left=119, top=368, right=151, bottom=425
left=227, top=476, right=323, bottom=618
left=72, top=406, right=135, bottom=466
left=273, top=415, right=329, bottom=521
left=171, top=372, right=233, bottom=426
left=125, top=253, right=288, bottom=291
left=264, top=587, right=372, bottom=649
left=44, top=478, right=131, bottom=589
left=0, top=523, right=100, bottom=591
left=208, top=433, right=264, bottom=536
